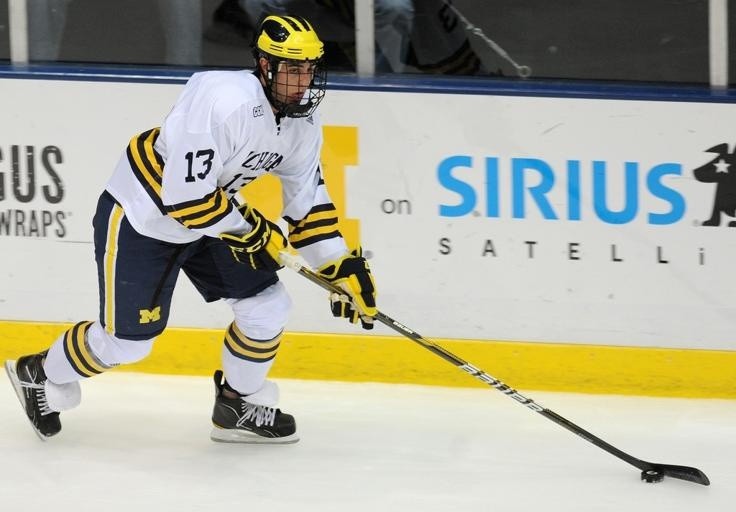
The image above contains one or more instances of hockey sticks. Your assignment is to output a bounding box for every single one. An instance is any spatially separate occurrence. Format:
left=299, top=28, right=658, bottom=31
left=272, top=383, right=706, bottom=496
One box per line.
left=279, top=251, right=710, bottom=487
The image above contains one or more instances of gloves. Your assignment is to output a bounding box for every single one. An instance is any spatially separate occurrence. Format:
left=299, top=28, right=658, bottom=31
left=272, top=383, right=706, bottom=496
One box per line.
left=314, top=244, right=379, bottom=330
left=219, top=199, right=288, bottom=272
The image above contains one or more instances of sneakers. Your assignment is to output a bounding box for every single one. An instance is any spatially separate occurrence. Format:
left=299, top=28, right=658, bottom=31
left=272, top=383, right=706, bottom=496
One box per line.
left=210, top=391, right=298, bottom=439
left=15, top=348, right=64, bottom=436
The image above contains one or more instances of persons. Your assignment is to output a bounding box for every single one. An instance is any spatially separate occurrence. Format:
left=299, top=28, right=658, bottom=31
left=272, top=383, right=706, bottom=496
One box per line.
left=201, top=0, right=508, bottom=78
left=13, top=15, right=380, bottom=439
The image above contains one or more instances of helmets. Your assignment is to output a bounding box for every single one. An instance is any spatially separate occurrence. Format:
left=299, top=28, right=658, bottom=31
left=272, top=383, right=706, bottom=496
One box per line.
left=248, top=14, right=328, bottom=118
